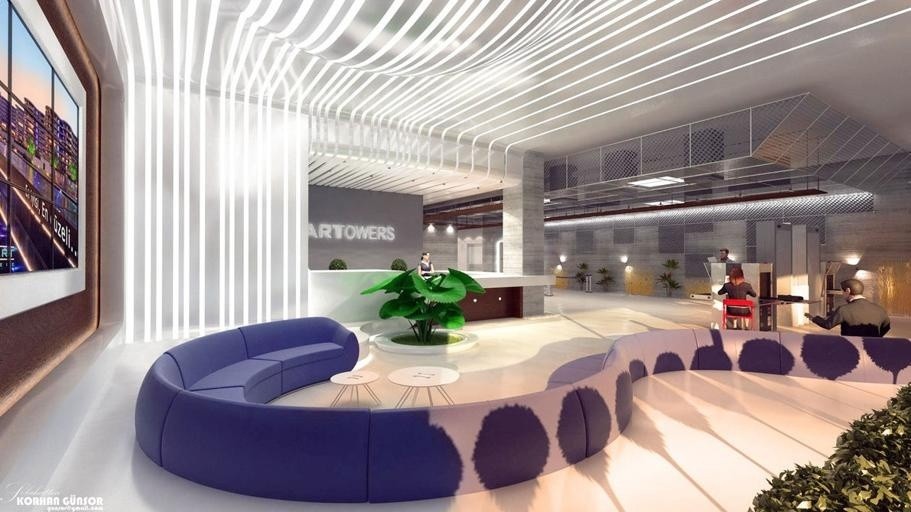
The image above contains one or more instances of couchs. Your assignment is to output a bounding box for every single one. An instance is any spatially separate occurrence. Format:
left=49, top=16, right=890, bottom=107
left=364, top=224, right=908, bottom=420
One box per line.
left=134, top=315, right=911, bottom=503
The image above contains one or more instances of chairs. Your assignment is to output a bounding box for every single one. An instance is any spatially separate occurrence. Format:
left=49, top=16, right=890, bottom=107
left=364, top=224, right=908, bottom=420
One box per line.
left=721, top=299, right=756, bottom=332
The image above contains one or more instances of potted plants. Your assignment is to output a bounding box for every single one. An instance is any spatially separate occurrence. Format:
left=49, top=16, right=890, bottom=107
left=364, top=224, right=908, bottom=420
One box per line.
left=574, top=261, right=617, bottom=292
left=656, top=259, right=683, bottom=296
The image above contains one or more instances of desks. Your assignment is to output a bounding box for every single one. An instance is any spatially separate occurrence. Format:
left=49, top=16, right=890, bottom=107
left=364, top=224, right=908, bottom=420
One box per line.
left=722, top=295, right=798, bottom=332
